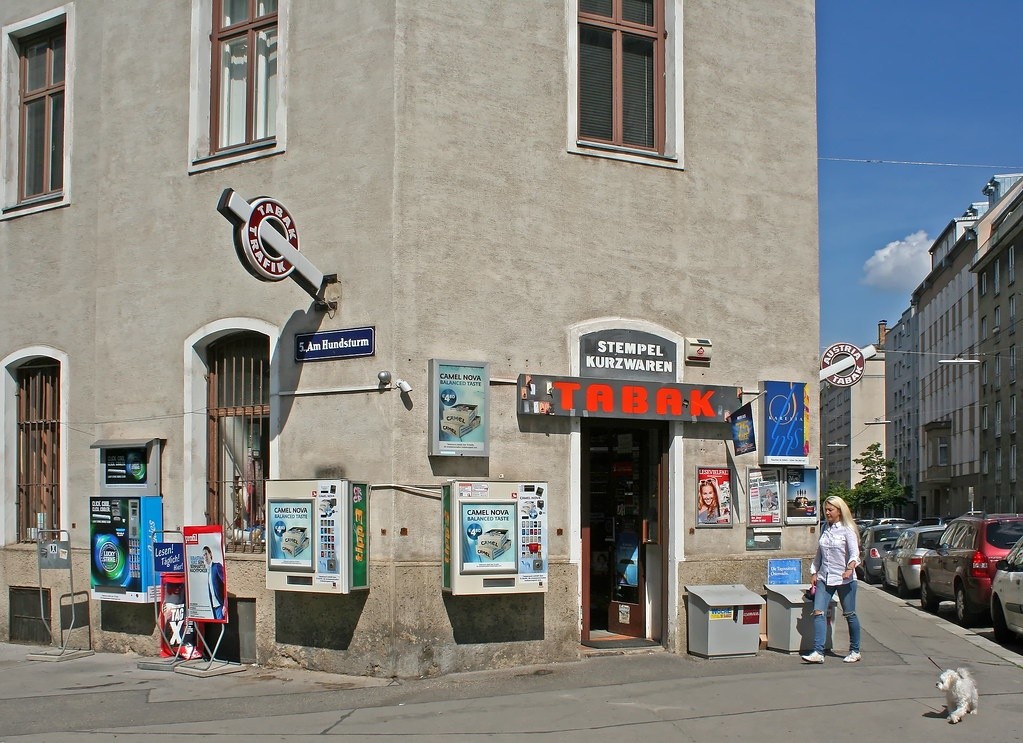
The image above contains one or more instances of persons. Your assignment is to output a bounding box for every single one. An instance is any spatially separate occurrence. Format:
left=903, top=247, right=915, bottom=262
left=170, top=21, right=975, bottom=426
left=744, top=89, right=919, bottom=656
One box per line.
left=801, top=495, right=861, bottom=663
left=202, top=546, right=226, bottom=619
left=597, top=554, right=609, bottom=568
left=766, top=489, right=777, bottom=510
left=698, top=479, right=720, bottom=524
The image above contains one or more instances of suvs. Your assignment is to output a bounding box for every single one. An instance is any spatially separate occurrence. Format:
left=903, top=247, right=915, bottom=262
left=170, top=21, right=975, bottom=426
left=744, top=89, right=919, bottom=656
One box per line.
left=918, top=509, right=1023, bottom=624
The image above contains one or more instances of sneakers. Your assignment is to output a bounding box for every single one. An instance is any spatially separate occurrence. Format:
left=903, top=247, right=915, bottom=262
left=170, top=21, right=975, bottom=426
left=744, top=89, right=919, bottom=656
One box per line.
left=802, top=651, right=824, bottom=664
left=843, top=651, right=861, bottom=662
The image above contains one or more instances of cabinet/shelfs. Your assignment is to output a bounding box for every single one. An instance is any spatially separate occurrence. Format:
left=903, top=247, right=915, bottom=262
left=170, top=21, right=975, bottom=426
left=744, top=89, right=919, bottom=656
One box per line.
left=590, top=447, right=609, bottom=494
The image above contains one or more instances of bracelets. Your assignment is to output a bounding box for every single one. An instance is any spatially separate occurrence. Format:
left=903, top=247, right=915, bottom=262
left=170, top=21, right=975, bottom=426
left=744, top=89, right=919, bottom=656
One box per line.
left=846, top=566, right=853, bottom=570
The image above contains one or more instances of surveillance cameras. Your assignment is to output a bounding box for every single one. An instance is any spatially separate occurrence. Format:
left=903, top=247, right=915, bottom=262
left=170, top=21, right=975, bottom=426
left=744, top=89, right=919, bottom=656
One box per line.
left=400, top=381, right=412, bottom=393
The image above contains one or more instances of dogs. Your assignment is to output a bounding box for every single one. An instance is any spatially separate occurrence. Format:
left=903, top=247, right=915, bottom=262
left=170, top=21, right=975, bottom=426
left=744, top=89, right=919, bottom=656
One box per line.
left=936, top=668, right=978, bottom=724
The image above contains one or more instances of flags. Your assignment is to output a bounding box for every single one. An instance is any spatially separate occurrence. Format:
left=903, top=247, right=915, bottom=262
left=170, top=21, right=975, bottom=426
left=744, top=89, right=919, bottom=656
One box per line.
left=730, top=402, right=757, bottom=456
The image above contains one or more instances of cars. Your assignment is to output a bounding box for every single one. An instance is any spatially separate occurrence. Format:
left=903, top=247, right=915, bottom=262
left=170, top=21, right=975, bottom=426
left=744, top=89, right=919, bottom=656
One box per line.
left=820, top=516, right=960, bottom=584
left=989, top=535, right=1023, bottom=648
left=882, top=525, right=947, bottom=597
